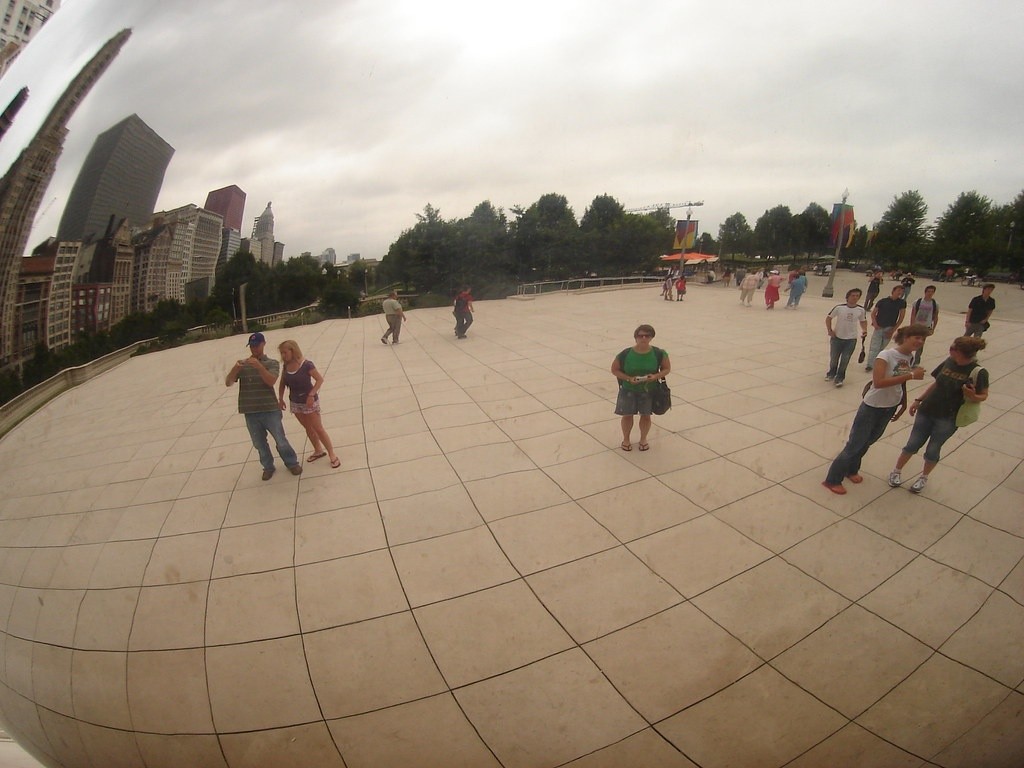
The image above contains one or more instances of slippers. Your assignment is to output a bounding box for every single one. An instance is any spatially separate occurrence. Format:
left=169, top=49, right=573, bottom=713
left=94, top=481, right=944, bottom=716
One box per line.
left=622, top=441, right=632, bottom=451
left=639, top=442, right=649, bottom=451
left=307, top=452, right=327, bottom=462
left=331, top=457, right=341, bottom=468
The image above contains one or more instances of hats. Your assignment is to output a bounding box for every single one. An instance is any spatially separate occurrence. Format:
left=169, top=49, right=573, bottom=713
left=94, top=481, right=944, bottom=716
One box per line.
left=245, top=333, right=265, bottom=347
left=770, top=270, right=778, bottom=275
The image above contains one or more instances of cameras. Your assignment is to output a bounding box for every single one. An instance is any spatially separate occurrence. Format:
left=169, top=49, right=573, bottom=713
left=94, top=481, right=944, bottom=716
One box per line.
left=963, top=376, right=974, bottom=390
left=635, top=376, right=647, bottom=381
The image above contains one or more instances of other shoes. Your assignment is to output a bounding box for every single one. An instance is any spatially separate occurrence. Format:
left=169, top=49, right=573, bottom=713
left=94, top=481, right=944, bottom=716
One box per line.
left=290, top=465, right=303, bottom=475
left=822, top=481, right=847, bottom=494
left=834, top=380, right=843, bottom=386
left=262, top=470, right=273, bottom=480
left=381, top=338, right=388, bottom=344
left=824, top=374, right=835, bottom=380
left=847, top=474, right=863, bottom=484
left=865, top=366, right=872, bottom=371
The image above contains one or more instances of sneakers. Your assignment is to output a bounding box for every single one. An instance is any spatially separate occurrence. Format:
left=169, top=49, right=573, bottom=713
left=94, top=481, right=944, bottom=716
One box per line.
left=889, top=470, right=901, bottom=487
left=908, top=475, right=928, bottom=493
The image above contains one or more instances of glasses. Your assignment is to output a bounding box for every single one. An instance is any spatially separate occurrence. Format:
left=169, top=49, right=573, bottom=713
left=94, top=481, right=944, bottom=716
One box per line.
left=637, top=335, right=652, bottom=339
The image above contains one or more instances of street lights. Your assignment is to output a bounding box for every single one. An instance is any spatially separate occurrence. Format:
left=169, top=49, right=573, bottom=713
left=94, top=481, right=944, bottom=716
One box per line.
left=1006, top=220, right=1016, bottom=250
left=679, top=205, right=694, bottom=276
left=821, top=188, right=849, bottom=297
left=322, top=267, right=327, bottom=288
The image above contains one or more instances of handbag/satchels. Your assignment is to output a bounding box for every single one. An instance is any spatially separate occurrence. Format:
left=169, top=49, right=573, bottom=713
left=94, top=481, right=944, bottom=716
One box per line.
left=955, top=368, right=980, bottom=427
left=652, top=349, right=671, bottom=415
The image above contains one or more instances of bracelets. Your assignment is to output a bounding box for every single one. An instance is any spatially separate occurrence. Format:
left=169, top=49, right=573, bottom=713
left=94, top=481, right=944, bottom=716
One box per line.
left=910, top=372, right=914, bottom=379
left=308, top=394, right=315, bottom=397
left=915, top=399, right=922, bottom=402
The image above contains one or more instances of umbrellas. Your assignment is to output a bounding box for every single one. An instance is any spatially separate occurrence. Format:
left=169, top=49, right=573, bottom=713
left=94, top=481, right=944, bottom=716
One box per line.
left=940, top=260, right=960, bottom=267
left=818, top=255, right=835, bottom=264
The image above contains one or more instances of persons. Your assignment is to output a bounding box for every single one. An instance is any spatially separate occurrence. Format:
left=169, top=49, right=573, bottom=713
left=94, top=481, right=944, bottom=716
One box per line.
left=813, top=264, right=832, bottom=276
left=380, top=291, right=407, bottom=345
left=964, top=284, right=995, bottom=337
left=946, top=267, right=955, bottom=279
left=888, top=335, right=988, bottom=493
left=901, top=272, right=915, bottom=296
left=910, top=285, right=939, bottom=366
left=864, top=274, right=880, bottom=310
left=723, top=266, right=807, bottom=309
left=224, top=332, right=303, bottom=480
left=822, top=323, right=932, bottom=495
left=276, top=338, right=341, bottom=469
left=825, top=288, right=867, bottom=387
left=888, top=268, right=903, bottom=280
left=611, top=324, right=671, bottom=452
left=964, top=266, right=969, bottom=280
left=660, top=273, right=686, bottom=301
left=453, top=284, right=475, bottom=339
left=865, top=285, right=907, bottom=370
